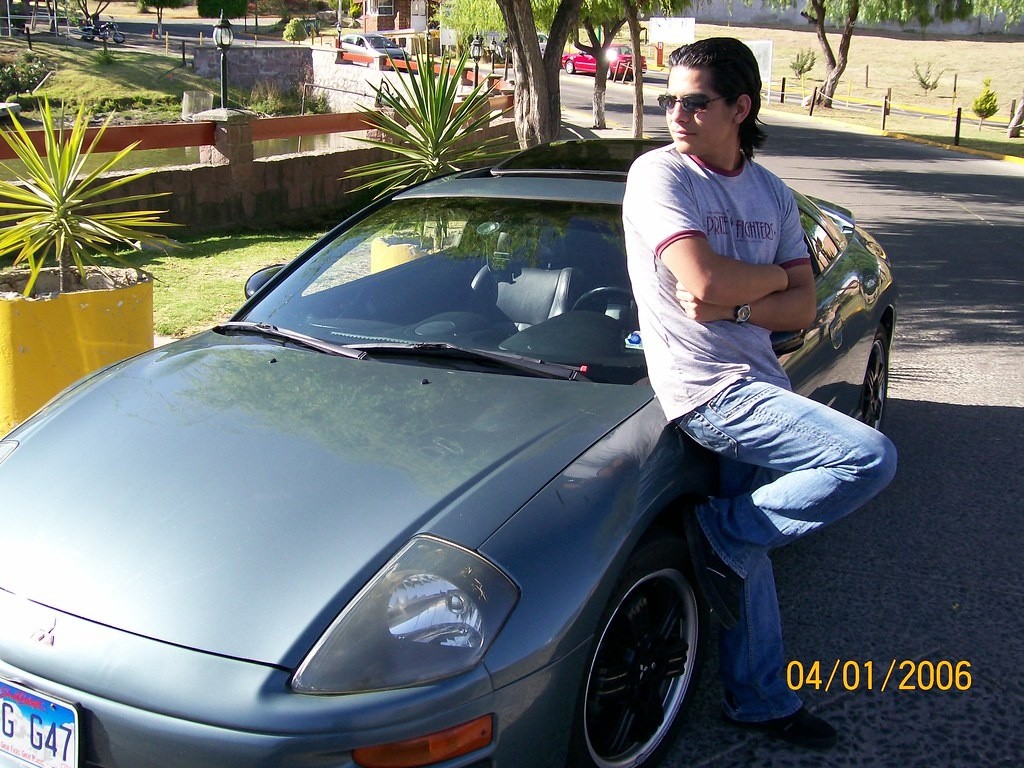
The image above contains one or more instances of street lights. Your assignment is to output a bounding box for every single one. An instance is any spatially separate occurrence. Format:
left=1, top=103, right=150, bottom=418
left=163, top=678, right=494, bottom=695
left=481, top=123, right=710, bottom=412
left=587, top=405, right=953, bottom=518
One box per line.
left=472, top=30, right=484, bottom=90
left=212, top=8, right=234, bottom=109
left=490, top=36, right=497, bottom=74
left=335, top=21, right=342, bottom=48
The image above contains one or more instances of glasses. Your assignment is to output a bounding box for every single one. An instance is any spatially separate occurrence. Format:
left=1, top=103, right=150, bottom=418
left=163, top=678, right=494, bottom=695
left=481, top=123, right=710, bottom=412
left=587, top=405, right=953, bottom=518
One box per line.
left=657, top=94, right=726, bottom=113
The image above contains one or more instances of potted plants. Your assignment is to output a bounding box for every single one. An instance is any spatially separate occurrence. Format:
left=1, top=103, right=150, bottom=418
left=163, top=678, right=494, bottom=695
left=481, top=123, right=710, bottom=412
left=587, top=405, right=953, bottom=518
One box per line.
left=0, top=89, right=191, bottom=437
left=333, top=22, right=539, bottom=278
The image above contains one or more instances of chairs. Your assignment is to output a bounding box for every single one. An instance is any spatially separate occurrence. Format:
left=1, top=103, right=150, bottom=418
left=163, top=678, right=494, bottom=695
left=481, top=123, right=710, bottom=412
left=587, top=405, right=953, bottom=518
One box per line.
left=462, top=259, right=575, bottom=334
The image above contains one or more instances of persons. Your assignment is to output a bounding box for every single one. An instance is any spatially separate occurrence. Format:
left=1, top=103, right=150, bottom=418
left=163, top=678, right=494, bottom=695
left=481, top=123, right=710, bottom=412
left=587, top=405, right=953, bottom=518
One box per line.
left=623, top=38, right=898, bottom=750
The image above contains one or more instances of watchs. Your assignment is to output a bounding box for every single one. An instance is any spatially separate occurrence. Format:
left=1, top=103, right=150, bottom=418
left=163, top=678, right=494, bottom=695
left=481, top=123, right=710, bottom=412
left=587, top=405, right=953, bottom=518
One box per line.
left=734, top=304, right=751, bottom=324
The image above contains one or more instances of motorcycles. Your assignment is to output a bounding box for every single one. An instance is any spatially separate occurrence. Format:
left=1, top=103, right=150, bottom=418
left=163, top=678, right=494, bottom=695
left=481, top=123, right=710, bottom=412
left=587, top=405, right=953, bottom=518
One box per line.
left=81, top=13, right=125, bottom=43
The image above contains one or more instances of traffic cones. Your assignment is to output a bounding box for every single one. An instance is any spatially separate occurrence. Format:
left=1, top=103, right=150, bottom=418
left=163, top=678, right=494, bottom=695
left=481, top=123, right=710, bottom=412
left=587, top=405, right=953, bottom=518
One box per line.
left=151, top=28, right=157, bottom=39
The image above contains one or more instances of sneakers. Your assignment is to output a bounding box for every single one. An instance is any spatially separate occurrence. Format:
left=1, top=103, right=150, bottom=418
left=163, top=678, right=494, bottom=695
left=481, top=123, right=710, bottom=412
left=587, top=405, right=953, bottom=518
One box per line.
left=722, top=704, right=838, bottom=749
left=683, top=508, right=742, bottom=631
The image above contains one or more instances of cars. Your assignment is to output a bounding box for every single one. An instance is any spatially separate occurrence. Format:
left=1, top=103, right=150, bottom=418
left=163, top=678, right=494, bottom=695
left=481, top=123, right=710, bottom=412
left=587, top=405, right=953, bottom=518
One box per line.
left=562, top=44, right=647, bottom=81
left=498, top=32, right=548, bottom=60
left=0, top=139, right=899, bottom=767
left=330, top=33, right=411, bottom=71
